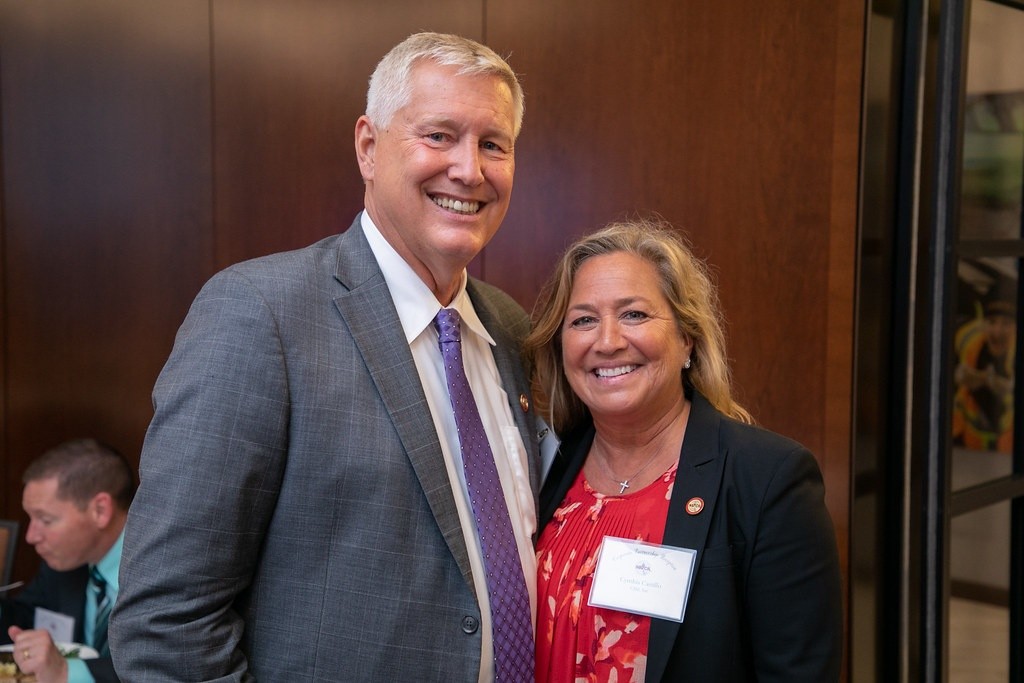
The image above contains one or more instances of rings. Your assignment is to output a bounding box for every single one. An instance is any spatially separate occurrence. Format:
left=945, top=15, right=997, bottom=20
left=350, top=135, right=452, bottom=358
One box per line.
left=23, top=650, right=29, bottom=659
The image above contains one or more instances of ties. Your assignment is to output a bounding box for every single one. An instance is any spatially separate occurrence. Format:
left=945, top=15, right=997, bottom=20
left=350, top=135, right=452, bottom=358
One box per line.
left=433, top=308, right=534, bottom=683
left=88, top=565, right=112, bottom=658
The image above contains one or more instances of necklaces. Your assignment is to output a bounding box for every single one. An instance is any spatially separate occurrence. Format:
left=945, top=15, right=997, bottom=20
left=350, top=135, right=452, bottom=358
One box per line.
left=592, top=434, right=659, bottom=494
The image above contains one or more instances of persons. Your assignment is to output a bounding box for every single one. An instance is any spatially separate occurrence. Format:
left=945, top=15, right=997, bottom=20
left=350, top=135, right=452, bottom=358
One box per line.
left=526, top=218, right=842, bottom=683
left=108, top=33, right=541, bottom=683
left=0, top=437, right=139, bottom=683
